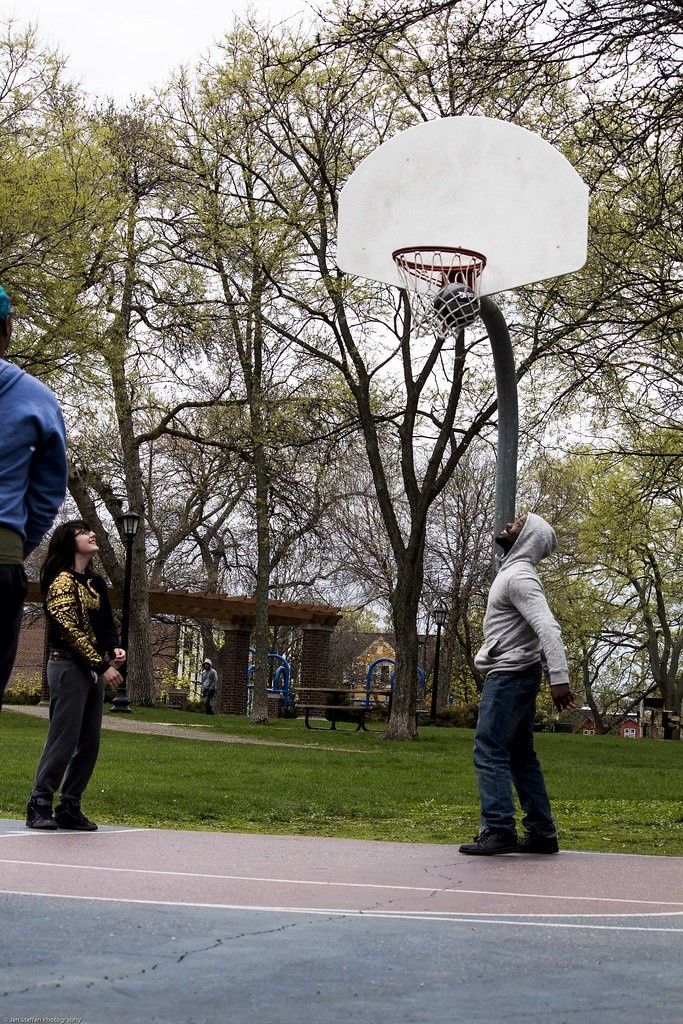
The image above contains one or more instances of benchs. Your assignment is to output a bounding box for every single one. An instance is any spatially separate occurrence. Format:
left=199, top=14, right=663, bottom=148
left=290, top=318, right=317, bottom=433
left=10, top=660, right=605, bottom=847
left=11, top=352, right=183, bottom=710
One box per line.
left=293, top=686, right=392, bottom=731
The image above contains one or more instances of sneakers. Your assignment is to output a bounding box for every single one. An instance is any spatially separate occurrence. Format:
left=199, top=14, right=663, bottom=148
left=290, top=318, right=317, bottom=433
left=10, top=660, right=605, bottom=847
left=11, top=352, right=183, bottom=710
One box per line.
left=54, top=801, right=98, bottom=830
left=459, top=829, right=518, bottom=854
left=25, top=797, right=57, bottom=829
left=510, top=832, right=559, bottom=854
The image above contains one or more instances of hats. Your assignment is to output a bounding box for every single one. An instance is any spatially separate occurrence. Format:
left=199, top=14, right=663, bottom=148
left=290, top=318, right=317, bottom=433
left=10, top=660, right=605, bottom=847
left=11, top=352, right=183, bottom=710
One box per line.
left=0, top=287, right=14, bottom=318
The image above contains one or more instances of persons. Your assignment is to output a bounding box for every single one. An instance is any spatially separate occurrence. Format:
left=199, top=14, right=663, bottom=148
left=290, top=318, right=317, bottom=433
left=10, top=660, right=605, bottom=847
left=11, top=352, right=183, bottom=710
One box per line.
left=456, top=511, right=580, bottom=856
left=0, top=287, right=70, bottom=724
left=24, top=519, right=128, bottom=830
left=200, top=659, right=218, bottom=716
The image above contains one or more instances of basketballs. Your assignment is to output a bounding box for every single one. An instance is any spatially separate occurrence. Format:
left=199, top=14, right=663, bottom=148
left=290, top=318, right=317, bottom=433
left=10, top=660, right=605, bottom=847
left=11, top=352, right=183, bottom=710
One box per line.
left=434, top=283, right=479, bottom=327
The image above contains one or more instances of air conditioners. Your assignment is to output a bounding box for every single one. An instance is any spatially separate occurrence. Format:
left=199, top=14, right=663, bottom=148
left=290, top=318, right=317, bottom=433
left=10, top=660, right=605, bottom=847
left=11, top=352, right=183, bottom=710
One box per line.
left=433, top=607, right=447, bottom=625
